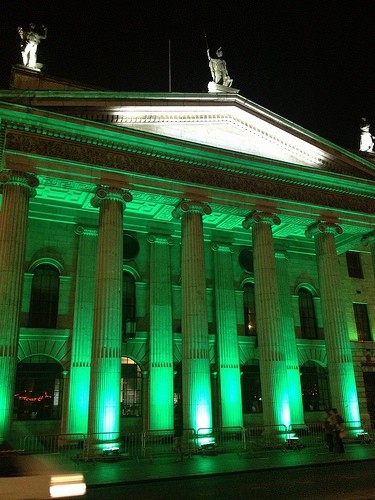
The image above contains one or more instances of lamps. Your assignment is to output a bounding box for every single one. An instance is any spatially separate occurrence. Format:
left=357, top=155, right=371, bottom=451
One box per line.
left=248, top=307, right=256, bottom=329
left=123, top=315, right=136, bottom=344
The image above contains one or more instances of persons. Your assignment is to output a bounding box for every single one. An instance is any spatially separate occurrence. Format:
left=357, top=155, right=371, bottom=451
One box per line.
left=321, top=409, right=334, bottom=456
left=329, top=408, right=347, bottom=455
